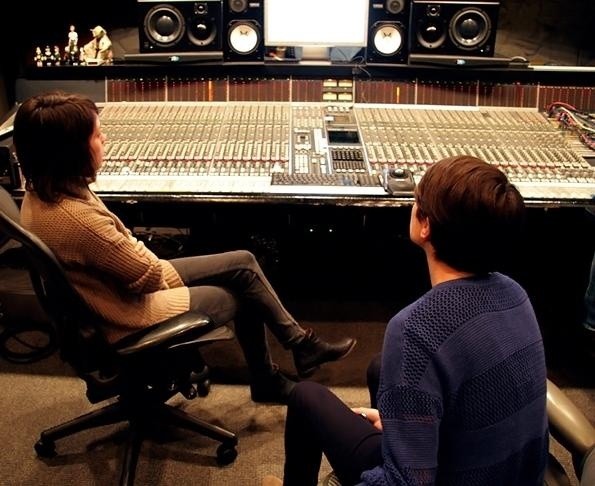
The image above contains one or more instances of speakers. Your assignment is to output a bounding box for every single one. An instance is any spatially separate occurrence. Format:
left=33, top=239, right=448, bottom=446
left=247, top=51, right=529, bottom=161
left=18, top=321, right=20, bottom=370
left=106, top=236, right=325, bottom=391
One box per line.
left=410, top=0, right=500, bottom=57
left=366, top=0, right=411, bottom=67
left=137, top=0, right=221, bottom=53
left=221, top=0, right=264, bottom=65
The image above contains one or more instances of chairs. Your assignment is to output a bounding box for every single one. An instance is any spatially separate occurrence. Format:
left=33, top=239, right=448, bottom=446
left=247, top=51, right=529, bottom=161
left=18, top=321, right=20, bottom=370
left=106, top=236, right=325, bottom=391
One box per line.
left=1, top=183, right=238, bottom=486
left=320, top=376, right=595, bottom=486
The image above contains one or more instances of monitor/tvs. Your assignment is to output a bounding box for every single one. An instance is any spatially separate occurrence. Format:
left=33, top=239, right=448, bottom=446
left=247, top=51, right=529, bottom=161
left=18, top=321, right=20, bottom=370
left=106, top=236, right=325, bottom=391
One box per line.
left=264, top=0, right=370, bottom=66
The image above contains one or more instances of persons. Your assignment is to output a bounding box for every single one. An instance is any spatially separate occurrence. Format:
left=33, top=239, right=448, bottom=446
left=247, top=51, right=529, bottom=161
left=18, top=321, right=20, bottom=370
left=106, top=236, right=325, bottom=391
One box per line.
left=12, top=89, right=356, bottom=403
left=260, top=155, right=549, bottom=486
left=32, top=24, right=111, bottom=66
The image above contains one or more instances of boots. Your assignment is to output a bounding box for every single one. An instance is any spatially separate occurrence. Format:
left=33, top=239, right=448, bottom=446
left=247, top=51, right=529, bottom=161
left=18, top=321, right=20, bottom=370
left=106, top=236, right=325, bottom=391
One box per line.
left=293, top=328, right=357, bottom=377
left=251, top=363, right=299, bottom=403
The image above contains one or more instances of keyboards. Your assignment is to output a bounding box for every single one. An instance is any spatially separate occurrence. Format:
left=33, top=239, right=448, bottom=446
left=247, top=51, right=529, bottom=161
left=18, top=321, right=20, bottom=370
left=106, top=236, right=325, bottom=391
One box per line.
left=271, top=172, right=382, bottom=187
